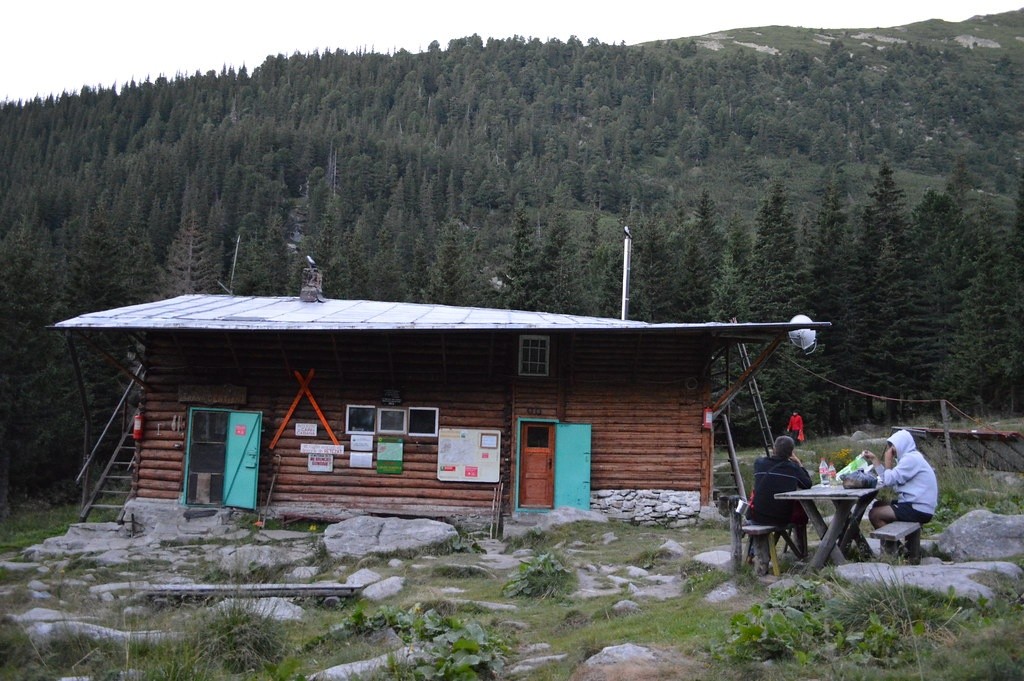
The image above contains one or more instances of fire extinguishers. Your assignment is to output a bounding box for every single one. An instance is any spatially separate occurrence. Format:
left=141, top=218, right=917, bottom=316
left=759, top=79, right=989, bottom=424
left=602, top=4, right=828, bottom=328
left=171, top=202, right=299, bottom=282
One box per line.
left=702, top=402, right=712, bottom=430
left=133, top=408, right=143, bottom=440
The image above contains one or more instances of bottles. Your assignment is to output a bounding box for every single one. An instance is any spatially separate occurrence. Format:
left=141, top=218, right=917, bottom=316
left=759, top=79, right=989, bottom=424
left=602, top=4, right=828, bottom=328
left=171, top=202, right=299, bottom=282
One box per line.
left=819, top=457, right=829, bottom=487
left=829, top=461, right=836, bottom=487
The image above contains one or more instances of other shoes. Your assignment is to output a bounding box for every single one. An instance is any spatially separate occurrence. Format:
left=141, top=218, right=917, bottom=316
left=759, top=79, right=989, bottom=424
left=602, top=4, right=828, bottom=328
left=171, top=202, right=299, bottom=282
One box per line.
left=899, top=545, right=911, bottom=558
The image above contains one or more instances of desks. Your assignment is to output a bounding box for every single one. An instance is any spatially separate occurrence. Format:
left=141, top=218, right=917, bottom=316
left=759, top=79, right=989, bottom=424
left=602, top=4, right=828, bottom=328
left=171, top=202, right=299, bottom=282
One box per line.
left=773, top=480, right=879, bottom=569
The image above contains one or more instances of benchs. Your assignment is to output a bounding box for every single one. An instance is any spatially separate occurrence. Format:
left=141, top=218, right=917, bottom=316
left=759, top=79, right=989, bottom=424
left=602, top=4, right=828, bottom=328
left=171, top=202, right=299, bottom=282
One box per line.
left=868, top=520, right=924, bottom=566
left=741, top=520, right=808, bottom=576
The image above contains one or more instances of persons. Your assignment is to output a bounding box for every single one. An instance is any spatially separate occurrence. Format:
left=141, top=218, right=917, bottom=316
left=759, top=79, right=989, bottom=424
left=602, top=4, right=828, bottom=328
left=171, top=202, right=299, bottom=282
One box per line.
left=748, top=436, right=812, bottom=564
left=864, top=429, right=937, bottom=558
left=787, top=411, right=803, bottom=445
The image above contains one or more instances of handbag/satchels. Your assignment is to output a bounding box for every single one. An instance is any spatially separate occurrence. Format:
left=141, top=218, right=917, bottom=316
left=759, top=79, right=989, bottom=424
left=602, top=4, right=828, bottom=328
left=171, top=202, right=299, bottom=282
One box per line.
left=744, top=489, right=757, bottom=521
left=797, top=430, right=804, bottom=443
left=834, top=449, right=874, bottom=481
left=840, top=469, right=878, bottom=490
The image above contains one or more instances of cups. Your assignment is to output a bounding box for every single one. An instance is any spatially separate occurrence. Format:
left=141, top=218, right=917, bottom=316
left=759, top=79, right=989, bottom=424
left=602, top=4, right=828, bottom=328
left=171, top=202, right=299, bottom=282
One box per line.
left=736, top=499, right=750, bottom=515
